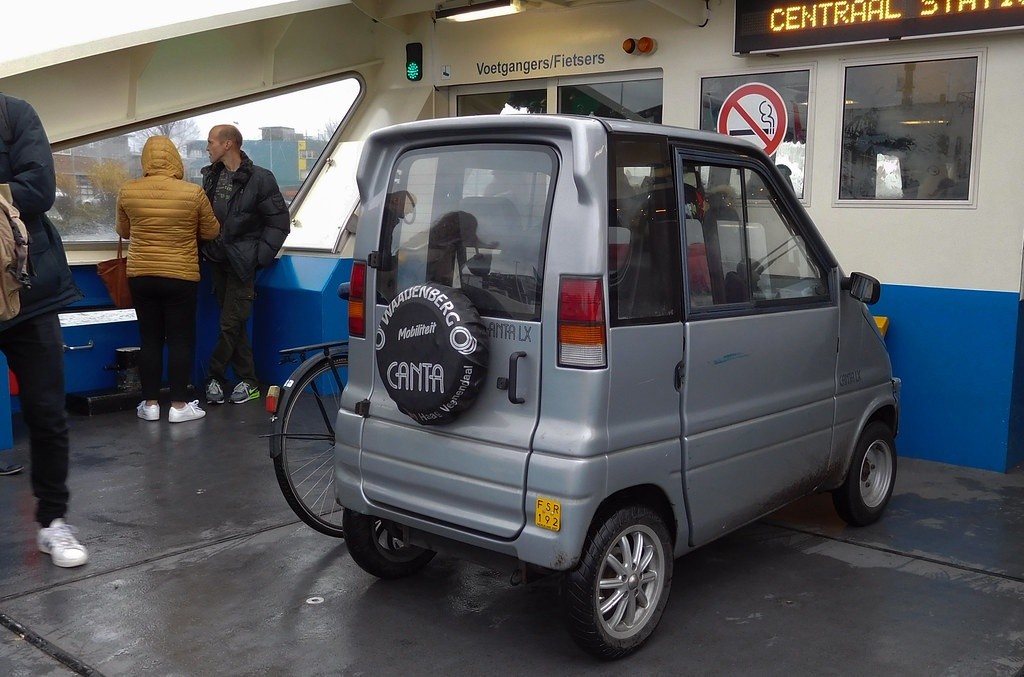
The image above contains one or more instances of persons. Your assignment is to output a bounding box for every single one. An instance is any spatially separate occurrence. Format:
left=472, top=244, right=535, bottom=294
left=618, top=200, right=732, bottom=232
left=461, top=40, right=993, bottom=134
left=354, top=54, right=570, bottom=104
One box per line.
left=639, top=159, right=705, bottom=219
left=0, top=92, right=94, bottom=568
left=199, top=126, right=291, bottom=406
left=708, top=185, right=741, bottom=219
left=115, top=135, right=219, bottom=423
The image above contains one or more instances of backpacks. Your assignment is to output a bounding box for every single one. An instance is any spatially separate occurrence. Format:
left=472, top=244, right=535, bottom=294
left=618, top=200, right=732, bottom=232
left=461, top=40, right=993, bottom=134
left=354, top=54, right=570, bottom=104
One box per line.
left=0, top=183, right=33, bottom=321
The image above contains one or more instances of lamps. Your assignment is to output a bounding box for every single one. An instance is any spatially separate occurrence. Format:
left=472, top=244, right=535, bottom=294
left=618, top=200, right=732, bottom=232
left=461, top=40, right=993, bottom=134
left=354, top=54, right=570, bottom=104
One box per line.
left=435, top=0, right=527, bottom=23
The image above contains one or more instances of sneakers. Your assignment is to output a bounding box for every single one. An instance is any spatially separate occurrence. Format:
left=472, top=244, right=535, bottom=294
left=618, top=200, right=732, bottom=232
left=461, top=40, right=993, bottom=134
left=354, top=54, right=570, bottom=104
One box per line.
left=136, top=399, right=160, bottom=420
left=206, top=379, right=225, bottom=404
left=0, top=463, right=24, bottom=475
left=38, top=518, right=89, bottom=568
left=228, top=381, right=260, bottom=403
left=168, top=400, right=206, bottom=422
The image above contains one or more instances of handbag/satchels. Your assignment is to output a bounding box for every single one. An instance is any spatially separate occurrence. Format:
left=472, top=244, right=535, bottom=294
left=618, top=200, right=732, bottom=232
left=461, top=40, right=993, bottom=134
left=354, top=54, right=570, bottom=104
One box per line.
left=97, top=236, right=134, bottom=308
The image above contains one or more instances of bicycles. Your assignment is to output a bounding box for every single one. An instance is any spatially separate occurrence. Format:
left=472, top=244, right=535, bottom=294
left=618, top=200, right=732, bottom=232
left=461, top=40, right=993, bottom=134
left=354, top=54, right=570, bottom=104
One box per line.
left=258, top=282, right=354, bottom=539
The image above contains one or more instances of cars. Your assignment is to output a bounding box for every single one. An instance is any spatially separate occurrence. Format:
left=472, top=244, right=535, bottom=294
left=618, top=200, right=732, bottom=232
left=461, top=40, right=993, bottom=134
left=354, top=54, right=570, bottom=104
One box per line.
left=43, top=205, right=63, bottom=223
left=55, top=187, right=69, bottom=200
left=82, top=191, right=109, bottom=209
left=333, top=114, right=902, bottom=663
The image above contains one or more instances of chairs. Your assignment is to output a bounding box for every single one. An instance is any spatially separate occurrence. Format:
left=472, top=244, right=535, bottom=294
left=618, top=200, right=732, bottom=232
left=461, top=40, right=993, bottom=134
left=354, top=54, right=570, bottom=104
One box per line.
left=457, top=195, right=543, bottom=323
left=608, top=183, right=726, bottom=310
